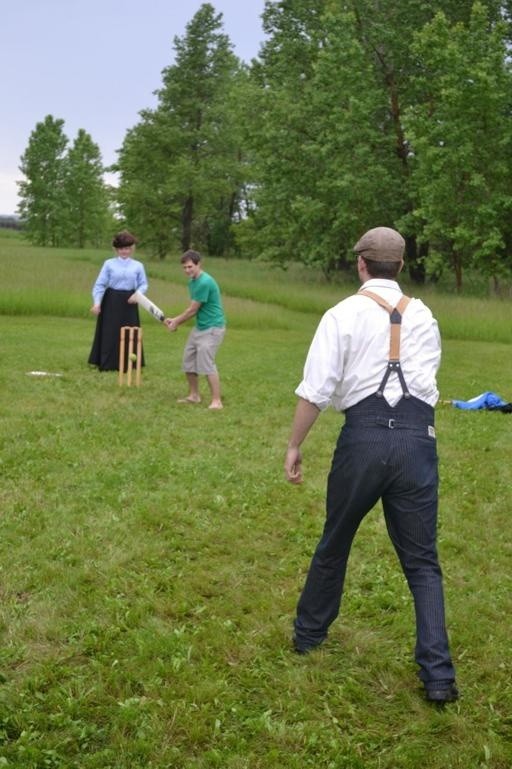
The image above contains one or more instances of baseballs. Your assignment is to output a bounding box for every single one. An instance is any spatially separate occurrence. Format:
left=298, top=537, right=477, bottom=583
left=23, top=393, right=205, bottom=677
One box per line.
left=130, top=354, right=136, bottom=361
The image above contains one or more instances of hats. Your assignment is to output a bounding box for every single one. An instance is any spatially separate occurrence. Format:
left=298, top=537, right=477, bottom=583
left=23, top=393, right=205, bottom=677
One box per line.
left=352, top=226, right=406, bottom=263
left=112, top=230, right=136, bottom=249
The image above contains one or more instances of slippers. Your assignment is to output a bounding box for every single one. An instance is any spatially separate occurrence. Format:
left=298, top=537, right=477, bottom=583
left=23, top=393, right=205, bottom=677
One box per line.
left=176, top=396, right=202, bottom=403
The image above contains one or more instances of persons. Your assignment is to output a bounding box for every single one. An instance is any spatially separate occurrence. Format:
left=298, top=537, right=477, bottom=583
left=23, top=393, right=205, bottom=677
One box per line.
left=88, top=230, right=148, bottom=373
left=284, top=227, right=458, bottom=704
left=162, top=249, right=227, bottom=410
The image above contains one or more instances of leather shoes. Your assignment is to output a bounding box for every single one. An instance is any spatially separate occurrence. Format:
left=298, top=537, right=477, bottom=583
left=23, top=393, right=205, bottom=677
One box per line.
left=426, top=686, right=459, bottom=703
